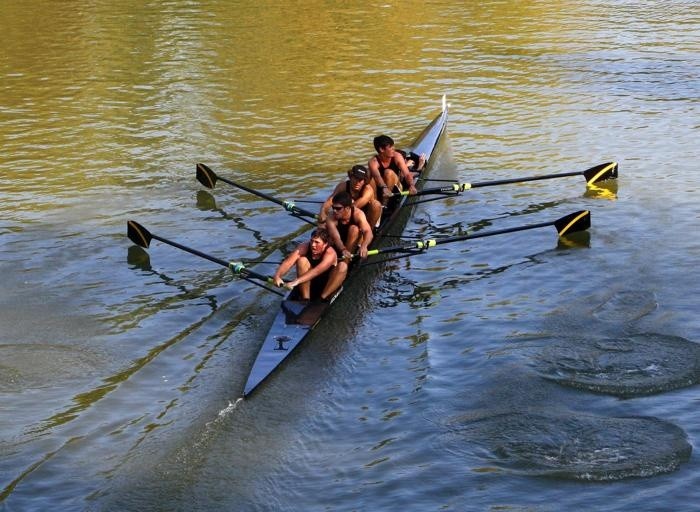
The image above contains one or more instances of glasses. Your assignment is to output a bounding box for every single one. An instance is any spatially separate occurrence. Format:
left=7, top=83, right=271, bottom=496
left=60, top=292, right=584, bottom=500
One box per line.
left=332, top=206, right=343, bottom=211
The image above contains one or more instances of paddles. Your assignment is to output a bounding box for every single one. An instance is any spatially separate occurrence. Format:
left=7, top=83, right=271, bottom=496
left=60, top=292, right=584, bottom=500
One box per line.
left=341, top=210, right=590, bottom=259
left=385, top=162, right=618, bottom=200
left=195, top=164, right=317, bottom=219
left=127, top=220, right=295, bottom=292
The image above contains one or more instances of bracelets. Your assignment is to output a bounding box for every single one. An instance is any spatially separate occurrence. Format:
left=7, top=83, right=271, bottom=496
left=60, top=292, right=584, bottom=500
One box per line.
left=340, top=248, right=347, bottom=252
left=381, top=186, right=389, bottom=188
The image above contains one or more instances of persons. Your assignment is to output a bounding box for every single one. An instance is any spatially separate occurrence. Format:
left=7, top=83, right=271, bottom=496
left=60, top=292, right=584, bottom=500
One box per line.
left=394, top=148, right=426, bottom=192
left=272, top=228, right=348, bottom=302
left=324, top=191, right=374, bottom=264
left=319, top=164, right=382, bottom=251
left=367, top=134, right=417, bottom=231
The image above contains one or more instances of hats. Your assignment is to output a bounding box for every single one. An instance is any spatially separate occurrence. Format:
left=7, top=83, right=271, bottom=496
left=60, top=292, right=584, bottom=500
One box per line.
left=352, top=165, right=366, bottom=179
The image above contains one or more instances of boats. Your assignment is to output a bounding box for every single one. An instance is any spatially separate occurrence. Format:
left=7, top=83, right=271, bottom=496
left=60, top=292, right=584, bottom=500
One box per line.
left=237, top=91, right=454, bottom=398
